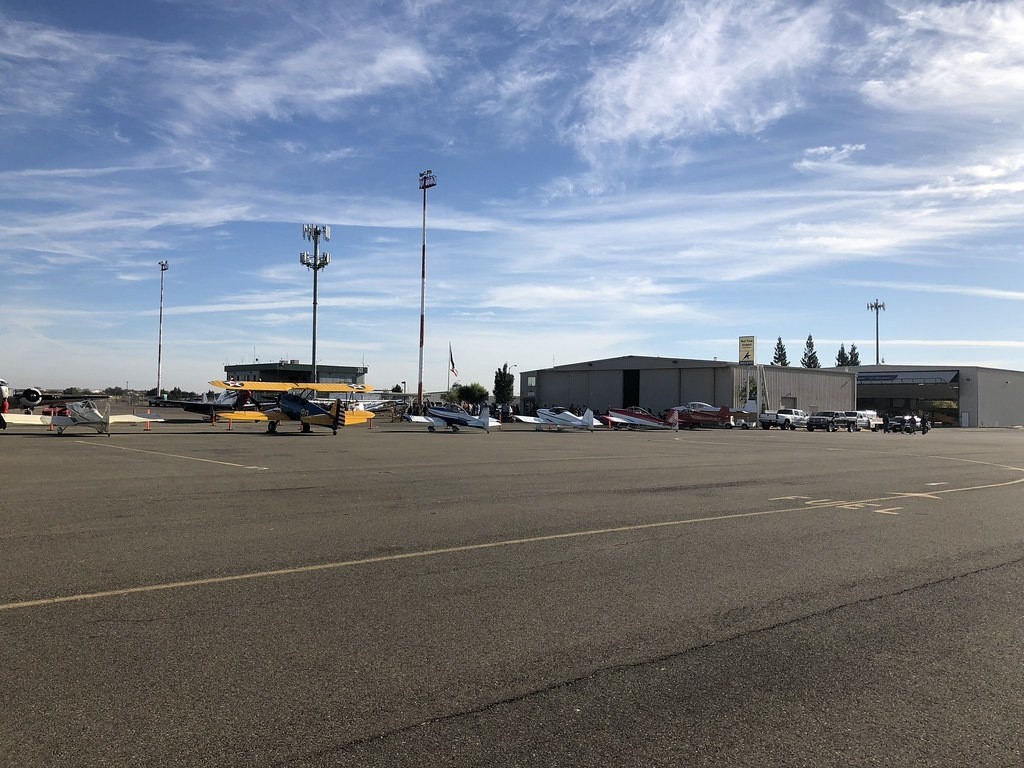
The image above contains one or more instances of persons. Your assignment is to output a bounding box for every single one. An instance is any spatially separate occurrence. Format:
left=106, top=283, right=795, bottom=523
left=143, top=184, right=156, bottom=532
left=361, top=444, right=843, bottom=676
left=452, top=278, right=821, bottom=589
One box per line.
left=642, top=407, right=670, bottom=420
left=525, top=402, right=611, bottom=420
left=879, top=412, right=928, bottom=435
left=393, top=401, right=520, bottom=423
left=931, top=417, right=936, bottom=428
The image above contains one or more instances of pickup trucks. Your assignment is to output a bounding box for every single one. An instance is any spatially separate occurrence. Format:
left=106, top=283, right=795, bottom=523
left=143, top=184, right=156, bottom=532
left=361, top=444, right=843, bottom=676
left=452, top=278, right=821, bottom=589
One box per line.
left=807, top=410, right=931, bottom=432
left=148, top=396, right=166, bottom=407
left=759, top=409, right=810, bottom=430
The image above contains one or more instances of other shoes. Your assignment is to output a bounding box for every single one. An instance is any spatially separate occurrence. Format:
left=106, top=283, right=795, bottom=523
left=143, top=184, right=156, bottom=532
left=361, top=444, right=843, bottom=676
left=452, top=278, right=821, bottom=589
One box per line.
left=4, top=423, right=8, bottom=430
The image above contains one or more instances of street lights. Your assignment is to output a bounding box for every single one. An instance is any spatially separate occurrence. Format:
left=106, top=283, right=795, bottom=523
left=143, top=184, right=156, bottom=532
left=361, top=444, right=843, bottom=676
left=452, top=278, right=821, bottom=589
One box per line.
left=418, top=169, right=437, bottom=416
left=300, top=224, right=331, bottom=399
left=157, top=259, right=169, bottom=396
left=508, top=364, right=517, bottom=406
left=401, top=381, right=406, bottom=393
left=867, top=299, right=885, bottom=365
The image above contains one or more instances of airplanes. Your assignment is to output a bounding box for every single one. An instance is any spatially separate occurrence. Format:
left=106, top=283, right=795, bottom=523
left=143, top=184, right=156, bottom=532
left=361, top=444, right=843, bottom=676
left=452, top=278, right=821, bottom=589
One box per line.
left=160, top=380, right=432, bottom=435
left=410, top=404, right=502, bottom=434
left=602, top=406, right=679, bottom=432
left=0, top=378, right=166, bottom=437
left=514, top=407, right=603, bottom=433
left=663, top=401, right=737, bottom=430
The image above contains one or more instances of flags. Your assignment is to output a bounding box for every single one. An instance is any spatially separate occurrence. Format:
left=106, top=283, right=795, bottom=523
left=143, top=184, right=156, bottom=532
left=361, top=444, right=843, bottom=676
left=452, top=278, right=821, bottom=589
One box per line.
left=448, top=341, right=458, bottom=377
